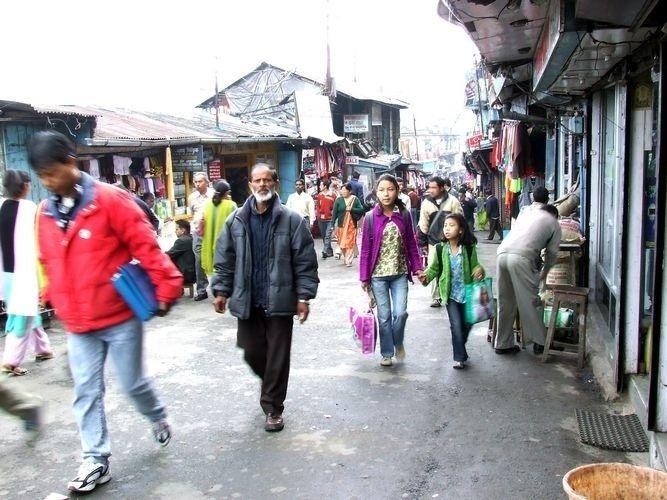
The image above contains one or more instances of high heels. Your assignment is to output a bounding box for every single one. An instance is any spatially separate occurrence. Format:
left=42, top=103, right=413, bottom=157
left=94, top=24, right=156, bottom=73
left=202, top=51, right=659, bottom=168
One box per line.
left=1, top=365, right=29, bottom=376
left=36, top=352, right=56, bottom=363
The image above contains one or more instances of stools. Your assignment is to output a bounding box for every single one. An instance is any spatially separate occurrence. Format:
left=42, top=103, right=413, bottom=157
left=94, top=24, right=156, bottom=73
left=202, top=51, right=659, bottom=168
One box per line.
left=542, top=286, right=590, bottom=368
left=486, top=298, right=525, bottom=351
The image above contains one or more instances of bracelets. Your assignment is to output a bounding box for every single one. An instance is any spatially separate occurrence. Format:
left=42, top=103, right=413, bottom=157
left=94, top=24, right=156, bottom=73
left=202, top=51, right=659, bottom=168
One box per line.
left=296, top=299, right=310, bottom=305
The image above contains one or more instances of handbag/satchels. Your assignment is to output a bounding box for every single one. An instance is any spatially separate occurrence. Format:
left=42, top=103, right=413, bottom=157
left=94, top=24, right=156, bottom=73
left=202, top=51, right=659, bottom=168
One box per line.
left=348, top=306, right=377, bottom=356
left=464, top=277, right=493, bottom=326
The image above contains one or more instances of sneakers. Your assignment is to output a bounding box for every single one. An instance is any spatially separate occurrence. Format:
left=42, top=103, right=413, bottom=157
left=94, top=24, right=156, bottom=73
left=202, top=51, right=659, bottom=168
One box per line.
left=67, top=461, right=112, bottom=492
left=380, top=356, right=393, bottom=367
left=264, top=410, right=285, bottom=433
left=194, top=294, right=208, bottom=301
left=494, top=346, right=521, bottom=354
left=430, top=303, right=441, bottom=307
left=533, top=342, right=565, bottom=355
left=452, top=361, right=465, bottom=370
left=151, top=418, right=172, bottom=447
left=395, top=346, right=406, bottom=360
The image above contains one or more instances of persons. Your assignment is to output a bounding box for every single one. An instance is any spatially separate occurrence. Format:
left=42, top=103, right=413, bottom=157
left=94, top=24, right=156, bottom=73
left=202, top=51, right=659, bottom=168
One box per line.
left=0, top=169, right=56, bottom=376
left=286, top=169, right=505, bottom=268
left=510, top=187, right=551, bottom=222
left=25, top=130, right=184, bottom=494
left=210, top=163, right=320, bottom=432
left=359, top=173, right=426, bottom=367
left=418, top=213, right=486, bottom=369
left=138, top=169, right=236, bottom=303
left=494, top=204, right=564, bottom=354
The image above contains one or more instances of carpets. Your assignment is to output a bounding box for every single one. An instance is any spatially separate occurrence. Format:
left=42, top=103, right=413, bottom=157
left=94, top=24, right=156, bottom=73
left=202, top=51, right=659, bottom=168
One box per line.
left=574, top=412, right=649, bottom=452
